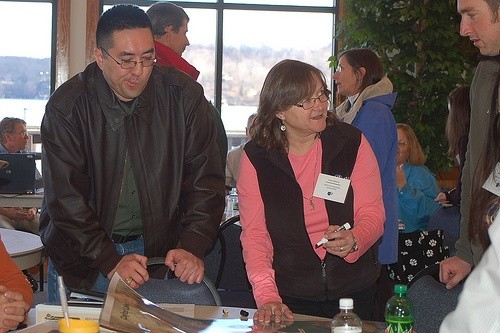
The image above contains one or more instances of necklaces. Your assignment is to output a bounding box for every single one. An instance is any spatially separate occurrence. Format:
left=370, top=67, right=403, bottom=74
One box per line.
left=294, top=136, right=324, bottom=209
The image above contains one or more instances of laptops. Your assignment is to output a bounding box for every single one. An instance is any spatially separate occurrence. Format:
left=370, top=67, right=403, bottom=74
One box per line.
left=0, top=154, right=37, bottom=195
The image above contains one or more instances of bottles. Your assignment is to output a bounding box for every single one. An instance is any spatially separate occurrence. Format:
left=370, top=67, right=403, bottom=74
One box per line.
left=331, top=297, right=362, bottom=333
left=385, top=284, right=415, bottom=333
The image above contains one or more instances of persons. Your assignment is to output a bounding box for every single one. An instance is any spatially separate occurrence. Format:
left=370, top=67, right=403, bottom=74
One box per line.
left=436, top=84, right=464, bottom=208
left=434, top=0, right=500, bottom=290
left=223, top=114, right=262, bottom=189
left=0, top=233, right=34, bottom=333
left=35, top=3, right=230, bottom=314
left=0, top=116, right=50, bottom=293
left=330, top=48, right=398, bottom=322
left=238, top=58, right=386, bottom=324
left=128, top=1, right=202, bottom=82
left=394, top=121, right=453, bottom=291
left=440, top=66, right=500, bottom=332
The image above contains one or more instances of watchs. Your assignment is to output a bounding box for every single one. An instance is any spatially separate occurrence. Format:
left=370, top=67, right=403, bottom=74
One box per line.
left=352, top=234, right=358, bottom=251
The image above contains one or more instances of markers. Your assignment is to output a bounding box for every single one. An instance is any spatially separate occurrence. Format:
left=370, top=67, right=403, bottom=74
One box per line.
left=315, top=222, right=352, bottom=248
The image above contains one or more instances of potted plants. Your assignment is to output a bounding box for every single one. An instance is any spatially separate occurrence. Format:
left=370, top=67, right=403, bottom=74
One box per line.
left=330, top=0, right=480, bottom=189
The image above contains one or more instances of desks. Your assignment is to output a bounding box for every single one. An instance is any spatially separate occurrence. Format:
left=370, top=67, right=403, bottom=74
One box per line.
left=156, top=302, right=385, bottom=333
left=0, top=194, right=44, bottom=208
left=0, top=228, right=44, bottom=293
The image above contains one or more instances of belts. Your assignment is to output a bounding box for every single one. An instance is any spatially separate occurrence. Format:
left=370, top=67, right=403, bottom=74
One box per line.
left=111, top=232, right=144, bottom=244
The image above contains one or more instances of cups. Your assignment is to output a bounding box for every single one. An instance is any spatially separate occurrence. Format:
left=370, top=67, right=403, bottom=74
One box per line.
left=226, top=194, right=240, bottom=224
left=59, top=317, right=99, bottom=333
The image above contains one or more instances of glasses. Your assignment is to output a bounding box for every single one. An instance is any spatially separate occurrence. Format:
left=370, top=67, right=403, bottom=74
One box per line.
left=99, top=46, right=157, bottom=70
left=335, top=64, right=342, bottom=73
left=293, top=88, right=332, bottom=110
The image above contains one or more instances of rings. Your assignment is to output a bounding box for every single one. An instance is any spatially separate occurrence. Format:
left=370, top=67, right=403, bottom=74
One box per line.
left=340, top=246, right=343, bottom=252
left=125, top=277, right=132, bottom=285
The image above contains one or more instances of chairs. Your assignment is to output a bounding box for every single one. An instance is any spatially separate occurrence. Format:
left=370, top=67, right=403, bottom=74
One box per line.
left=136, top=215, right=259, bottom=308
